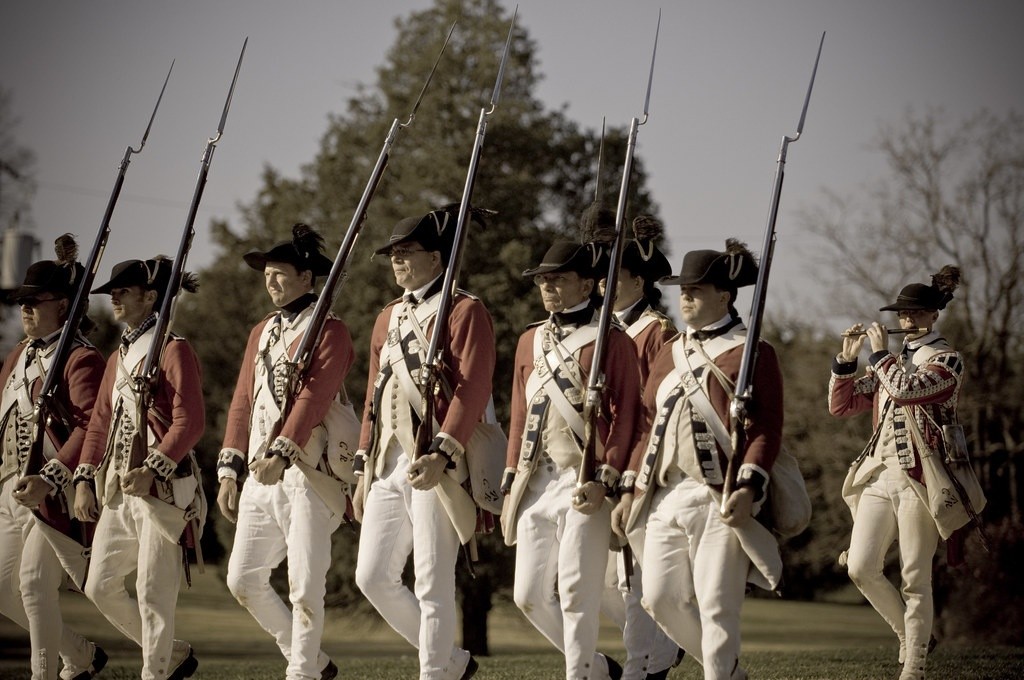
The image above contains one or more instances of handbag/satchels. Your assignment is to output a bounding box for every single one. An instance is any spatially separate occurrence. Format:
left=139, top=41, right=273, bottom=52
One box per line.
left=770, top=445, right=812, bottom=539
left=323, top=401, right=362, bottom=484
left=465, top=423, right=508, bottom=515
left=920, top=452, right=987, bottom=540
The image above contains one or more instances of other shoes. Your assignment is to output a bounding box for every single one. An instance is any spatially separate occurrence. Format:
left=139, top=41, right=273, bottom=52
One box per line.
left=460, top=656, right=478, bottom=680
left=72, top=647, right=108, bottom=680
left=605, top=654, right=623, bottom=680
left=320, top=660, right=338, bottom=680
left=645, top=647, right=685, bottom=680
left=167, top=648, right=198, bottom=680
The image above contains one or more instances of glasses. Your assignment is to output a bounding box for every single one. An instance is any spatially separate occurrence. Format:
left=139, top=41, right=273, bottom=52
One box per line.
left=533, top=274, right=566, bottom=286
left=389, top=248, right=425, bottom=255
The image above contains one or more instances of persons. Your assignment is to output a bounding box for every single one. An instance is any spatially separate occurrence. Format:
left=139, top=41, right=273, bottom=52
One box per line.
left=828, top=264, right=965, bottom=680
left=352, top=211, right=496, bottom=680
left=74, top=254, right=205, bottom=680
left=217, top=220, right=352, bottom=680
left=610, top=237, right=783, bottom=680
left=0, top=260, right=107, bottom=680
left=500, top=239, right=685, bottom=680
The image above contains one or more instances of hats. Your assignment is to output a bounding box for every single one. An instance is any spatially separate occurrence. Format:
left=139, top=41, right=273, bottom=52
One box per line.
left=375, top=210, right=452, bottom=255
left=90, top=260, right=179, bottom=303
left=658, top=238, right=759, bottom=288
left=621, top=216, right=672, bottom=282
left=243, top=223, right=333, bottom=276
left=522, top=201, right=617, bottom=275
left=879, top=265, right=960, bottom=311
left=7, top=260, right=85, bottom=306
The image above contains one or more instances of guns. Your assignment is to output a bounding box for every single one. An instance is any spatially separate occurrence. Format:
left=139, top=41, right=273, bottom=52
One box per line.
left=263, top=14, right=460, bottom=480
left=414, top=4, right=526, bottom=476
left=19, top=58, right=175, bottom=495
left=573, top=7, right=663, bottom=506
left=721, top=30, right=831, bottom=522
left=126, top=33, right=251, bottom=475
